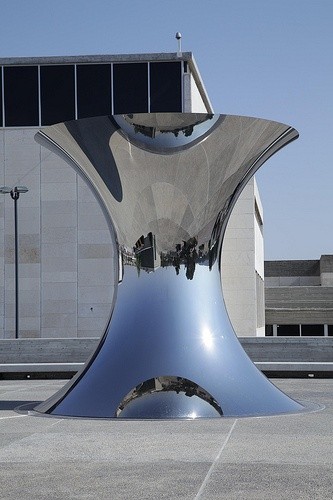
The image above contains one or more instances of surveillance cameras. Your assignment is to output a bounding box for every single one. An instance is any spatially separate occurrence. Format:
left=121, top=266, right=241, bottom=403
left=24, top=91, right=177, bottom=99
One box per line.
left=176, top=32, right=181, bottom=39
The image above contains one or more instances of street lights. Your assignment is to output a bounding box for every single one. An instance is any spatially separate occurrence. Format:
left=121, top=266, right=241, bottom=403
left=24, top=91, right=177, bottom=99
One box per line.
left=0, top=185, right=30, bottom=340
